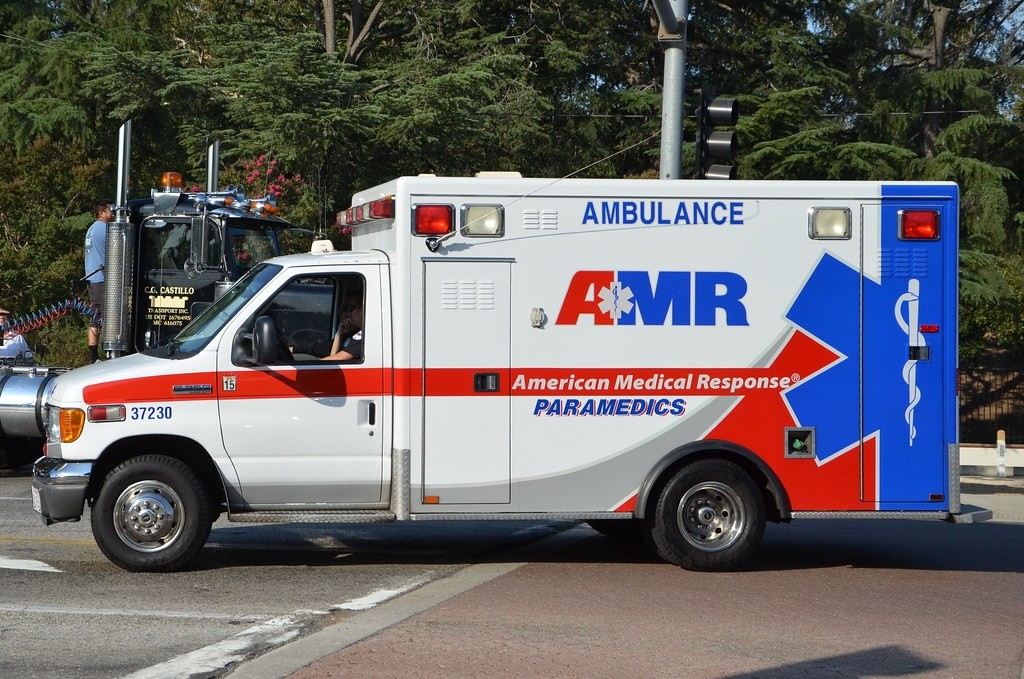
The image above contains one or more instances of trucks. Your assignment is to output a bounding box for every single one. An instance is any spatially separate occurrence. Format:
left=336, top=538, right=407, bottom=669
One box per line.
left=1, top=117, right=335, bottom=458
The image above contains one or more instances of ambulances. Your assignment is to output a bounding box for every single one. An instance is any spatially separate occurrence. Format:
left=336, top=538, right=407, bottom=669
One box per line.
left=31, top=172, right=962, bottom=576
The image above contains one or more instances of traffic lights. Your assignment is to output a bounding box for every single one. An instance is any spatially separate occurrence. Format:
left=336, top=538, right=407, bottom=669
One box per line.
left=694, top=85, right=740, bottom=181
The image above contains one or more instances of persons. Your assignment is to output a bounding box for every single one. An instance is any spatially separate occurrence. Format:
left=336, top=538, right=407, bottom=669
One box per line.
left=0, top=309, right=33, bottom=365
left=85, top=200, right=116, bottom=364
left=285, top=291, right=362, bottom=360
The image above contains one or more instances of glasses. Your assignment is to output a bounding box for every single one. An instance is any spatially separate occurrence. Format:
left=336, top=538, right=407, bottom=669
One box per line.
left=350, top=305, right=360, bottom=312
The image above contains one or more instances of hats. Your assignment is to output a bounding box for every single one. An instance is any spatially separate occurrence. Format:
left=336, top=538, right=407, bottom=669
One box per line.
left=0, top=308, right=10, bottom=313
